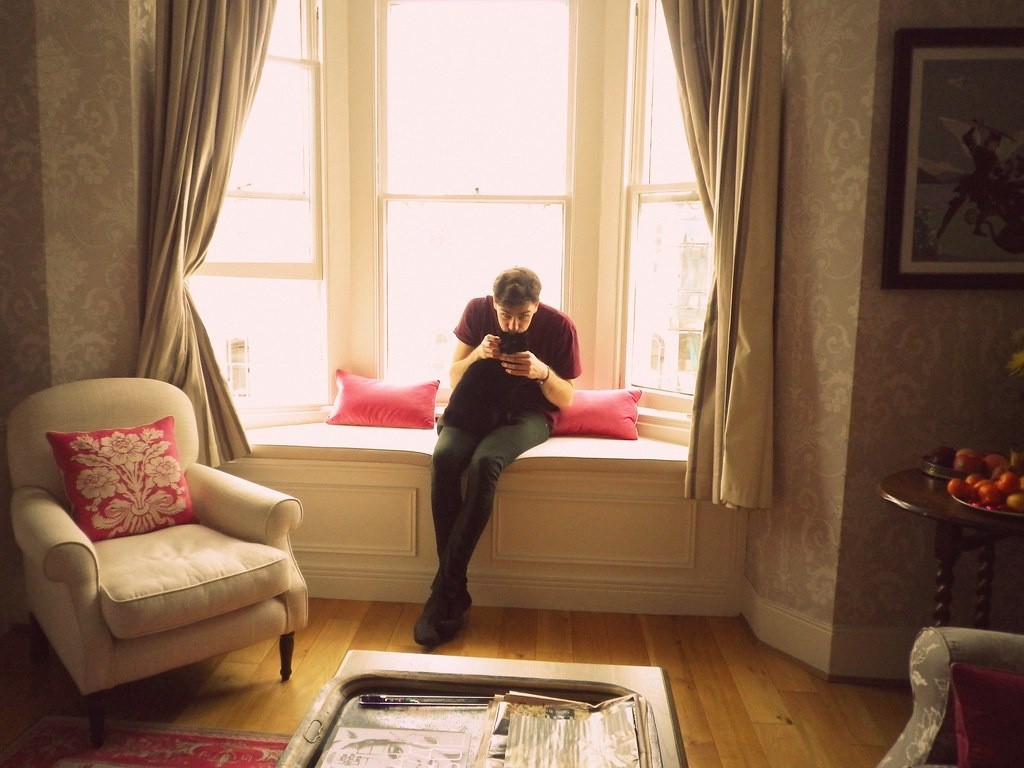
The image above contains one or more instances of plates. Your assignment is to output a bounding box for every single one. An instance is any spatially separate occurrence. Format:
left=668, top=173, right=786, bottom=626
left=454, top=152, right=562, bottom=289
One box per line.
left=920, top=455, right=967, bottom=479
left=952, top=493, right=1024, bottom=517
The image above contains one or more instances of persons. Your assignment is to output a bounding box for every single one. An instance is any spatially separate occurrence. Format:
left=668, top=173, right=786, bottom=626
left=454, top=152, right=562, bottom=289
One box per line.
left=412, top=268, right=583, bottom=648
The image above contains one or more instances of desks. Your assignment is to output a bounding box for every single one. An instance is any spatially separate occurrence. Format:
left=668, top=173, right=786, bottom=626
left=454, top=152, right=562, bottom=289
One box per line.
left=879, top=468, right=1024, bottom=632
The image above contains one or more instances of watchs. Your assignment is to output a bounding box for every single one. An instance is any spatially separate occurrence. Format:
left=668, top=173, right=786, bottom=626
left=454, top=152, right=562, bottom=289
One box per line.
left=535, top=365, right=550, bottom=385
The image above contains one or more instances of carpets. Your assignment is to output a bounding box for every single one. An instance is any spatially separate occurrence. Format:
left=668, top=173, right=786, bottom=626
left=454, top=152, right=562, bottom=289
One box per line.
left=0, top=715, right=292, bottom=768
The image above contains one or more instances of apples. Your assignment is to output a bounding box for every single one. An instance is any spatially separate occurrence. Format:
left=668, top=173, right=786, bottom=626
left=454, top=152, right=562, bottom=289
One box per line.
left=925, top=445, right=1024, bottom=513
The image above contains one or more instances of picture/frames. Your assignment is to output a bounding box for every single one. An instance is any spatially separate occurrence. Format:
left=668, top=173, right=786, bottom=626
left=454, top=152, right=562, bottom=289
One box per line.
left=879, top=26, right=1024, bottom=290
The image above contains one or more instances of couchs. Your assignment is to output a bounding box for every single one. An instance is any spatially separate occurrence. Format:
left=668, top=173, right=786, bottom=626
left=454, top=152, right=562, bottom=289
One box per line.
left=877, top=626, right=1024, bottom=768
left=4, top=376, right=310, bottom=748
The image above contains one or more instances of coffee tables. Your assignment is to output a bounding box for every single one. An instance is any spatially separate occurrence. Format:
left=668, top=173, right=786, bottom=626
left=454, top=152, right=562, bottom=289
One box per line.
left=274, top=646, right=688, bottom=768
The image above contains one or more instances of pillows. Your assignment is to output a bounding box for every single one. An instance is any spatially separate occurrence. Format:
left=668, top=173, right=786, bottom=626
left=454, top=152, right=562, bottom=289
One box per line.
left=550, top=387, right=643, bottom=440
left=47, top=414, right=199, bottom=542
left=950, top=661, right=1024, bottom=768
left=324, top=368, right=441, bottom=431
